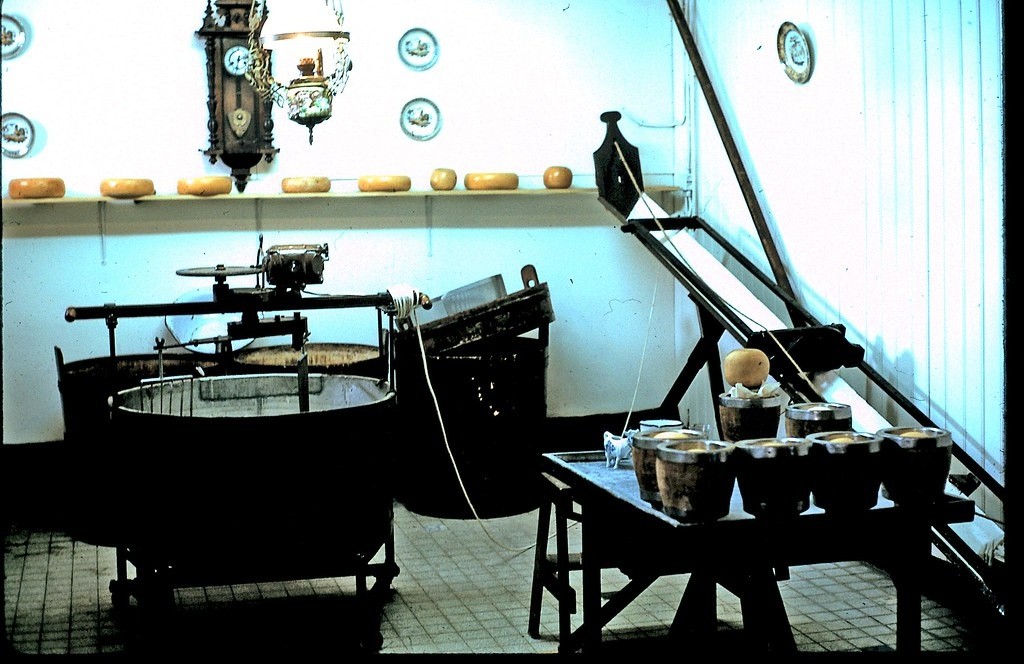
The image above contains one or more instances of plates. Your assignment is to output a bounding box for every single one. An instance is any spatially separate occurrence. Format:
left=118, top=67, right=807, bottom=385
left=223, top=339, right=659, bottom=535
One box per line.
left=400, top=97, right=442, bottom=141
left=0, top=14, right=25, bottom=59
left=778, top=22, right=812, bottom=85
left=397, top=28, right=440, bottom=70
left=0, top=113, right=34, bottom=158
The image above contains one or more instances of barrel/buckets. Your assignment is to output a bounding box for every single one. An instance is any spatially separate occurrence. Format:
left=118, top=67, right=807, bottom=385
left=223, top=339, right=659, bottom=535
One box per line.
left=391, top=328, right=553, bottom=519
left=878, top=426, right=953, bottom=504
left=629, top=430, right=703, bottom=500
left=105, top=372, right=393, bottom=573
left=736, top=438, right=811, bottom=516
left=55, top=348, right=233, bottom=437
left=785, top=403, right=852, bottom=439
left=233, top=342, right=383, bottom=378
left=807, top=431, right=885, bottom=512
left=657, top=441, right=735, bottom=520
left=719, top=392, right=781, bottom=442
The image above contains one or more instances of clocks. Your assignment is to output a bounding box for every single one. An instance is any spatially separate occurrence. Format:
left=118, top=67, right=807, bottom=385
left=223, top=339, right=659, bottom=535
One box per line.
left=196, top=0, right=281, bottom=194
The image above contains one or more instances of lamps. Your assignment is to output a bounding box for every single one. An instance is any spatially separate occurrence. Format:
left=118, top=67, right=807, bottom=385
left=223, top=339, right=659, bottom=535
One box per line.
left=243, top=0, right=353, bottom=146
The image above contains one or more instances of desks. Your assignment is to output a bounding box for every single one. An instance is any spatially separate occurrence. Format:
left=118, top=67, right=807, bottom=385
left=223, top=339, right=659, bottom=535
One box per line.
left=542, top=449, right=975, bottom=661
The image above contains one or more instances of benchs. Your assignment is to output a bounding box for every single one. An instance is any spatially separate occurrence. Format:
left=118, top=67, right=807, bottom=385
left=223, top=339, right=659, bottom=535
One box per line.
left=527, top=471, right=613, bottom=639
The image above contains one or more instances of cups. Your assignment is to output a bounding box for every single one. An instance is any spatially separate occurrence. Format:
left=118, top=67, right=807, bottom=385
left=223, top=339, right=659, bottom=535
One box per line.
left=639, top=419, right=683, bottom=432
